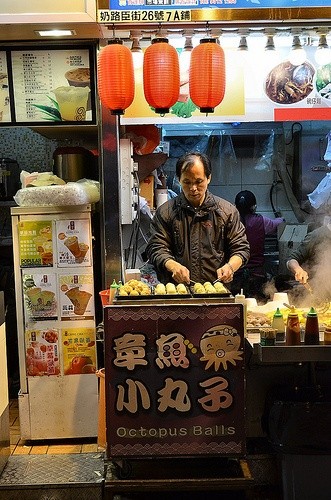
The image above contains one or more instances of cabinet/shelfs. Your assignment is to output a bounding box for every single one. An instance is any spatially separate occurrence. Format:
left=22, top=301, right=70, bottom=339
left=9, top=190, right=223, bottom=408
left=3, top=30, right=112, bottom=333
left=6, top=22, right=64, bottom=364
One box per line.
left=0, top=201, right=17, bottom=245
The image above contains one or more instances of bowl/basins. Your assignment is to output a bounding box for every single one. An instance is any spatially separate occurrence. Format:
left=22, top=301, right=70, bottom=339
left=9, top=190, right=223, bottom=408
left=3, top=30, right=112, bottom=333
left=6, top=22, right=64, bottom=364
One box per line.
left=263, top=58, right=315, bottom=108
left=99, top=288, right=110, bottom=305
left=313, top=73, right=331, bottom=107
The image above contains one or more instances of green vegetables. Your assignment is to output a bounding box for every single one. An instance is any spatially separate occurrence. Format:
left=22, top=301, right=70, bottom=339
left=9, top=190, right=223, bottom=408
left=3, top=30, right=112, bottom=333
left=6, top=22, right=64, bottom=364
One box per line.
left=316, top=64, right=331, bottom=92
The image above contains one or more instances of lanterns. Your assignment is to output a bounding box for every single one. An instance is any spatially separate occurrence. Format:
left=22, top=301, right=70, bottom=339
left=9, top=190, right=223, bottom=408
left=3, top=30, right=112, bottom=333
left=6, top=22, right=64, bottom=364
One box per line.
left=189, top=38, right=226, bottom=113
left=143, top=38, right=181, bottom=115
left=96, top=39, right=136, bottom=116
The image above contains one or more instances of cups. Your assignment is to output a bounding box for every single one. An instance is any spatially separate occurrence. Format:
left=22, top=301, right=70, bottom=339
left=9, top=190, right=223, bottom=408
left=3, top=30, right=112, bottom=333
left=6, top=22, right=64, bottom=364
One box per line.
left=41, top=252, right=53, bottom=264
left=41, top=290, right=55, bottom=310
left=28, top=342, right=55, bottom=370
left=260, top=337, right=275, bottom=346
left=64, top=236, right=81, bottom=257
left=26, top=286, right=43, bottom=309
left=65, top=287, right=80, bottom=306
left=65, top=68, right=91, bottom=111
left=51, top=86, right=89, bottom=121
left=75, top=243, right=89, bottom=264
left=324, top=327, right=331, bottom=345
left=74, top=291, right=92, bottom=315
left=41, top=329, right=58, bottom=346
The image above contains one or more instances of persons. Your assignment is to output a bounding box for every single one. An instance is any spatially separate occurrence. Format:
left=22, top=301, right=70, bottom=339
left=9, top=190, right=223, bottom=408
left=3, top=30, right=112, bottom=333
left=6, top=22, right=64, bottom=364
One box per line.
left=231, top=191, right=283, bottom=299
left=285, top=218, right=331, bottom=306
left=147, top=153, right=249, bottom=282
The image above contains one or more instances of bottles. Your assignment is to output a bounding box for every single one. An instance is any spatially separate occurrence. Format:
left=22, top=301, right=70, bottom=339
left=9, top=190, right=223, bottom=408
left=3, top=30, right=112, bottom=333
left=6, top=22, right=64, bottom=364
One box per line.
left=158, top=173, right=167, bottom=188
left=286, top=306, right=300, bottom=346
left=304, top=307, right=319, bottom=345
left=155, top=189, right=168, bottom=217
left=109, top=279, right=122, bottom=304
left=273, top=308, right=285, bottom=341
left=234, top=288, right=247, bottom=334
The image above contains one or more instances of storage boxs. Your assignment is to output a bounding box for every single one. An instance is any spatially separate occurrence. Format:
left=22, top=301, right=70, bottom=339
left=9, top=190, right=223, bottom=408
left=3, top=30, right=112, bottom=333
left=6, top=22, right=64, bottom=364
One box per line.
left=139, top=174, right=155, bottom=209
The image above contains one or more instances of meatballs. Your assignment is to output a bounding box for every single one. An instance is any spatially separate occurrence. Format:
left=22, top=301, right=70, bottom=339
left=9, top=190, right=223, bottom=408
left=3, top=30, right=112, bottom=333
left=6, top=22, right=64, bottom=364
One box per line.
left=118, top=279, right=227, bottom=296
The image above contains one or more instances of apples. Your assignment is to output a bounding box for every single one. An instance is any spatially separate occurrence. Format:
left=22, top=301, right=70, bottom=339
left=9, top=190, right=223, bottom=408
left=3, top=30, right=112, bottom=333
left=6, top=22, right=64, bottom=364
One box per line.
left=71, top=356, right=95, bottom=374
